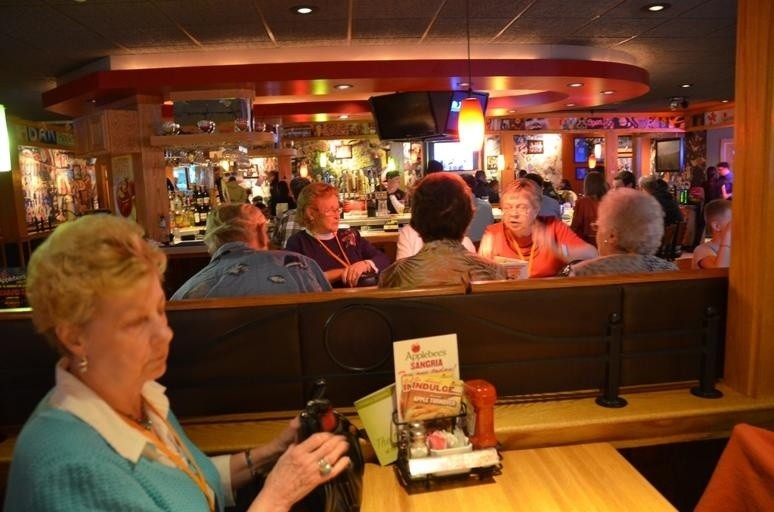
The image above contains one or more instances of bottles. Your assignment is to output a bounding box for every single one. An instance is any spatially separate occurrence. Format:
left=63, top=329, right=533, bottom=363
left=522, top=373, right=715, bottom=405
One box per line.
left=408, top=420, right=429, bottom=459
left=168, top=183, right=212, bottom=229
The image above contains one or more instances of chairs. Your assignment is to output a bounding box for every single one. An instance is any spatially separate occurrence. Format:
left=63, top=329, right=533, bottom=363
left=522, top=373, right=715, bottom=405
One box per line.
left=656, top=215, right=689, bottom=260
left=696, top=422, right=774, bottom=511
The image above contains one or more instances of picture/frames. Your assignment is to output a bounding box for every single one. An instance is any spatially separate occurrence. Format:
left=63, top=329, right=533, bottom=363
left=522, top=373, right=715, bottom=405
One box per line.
left=720, top=137, right=734, bottom=172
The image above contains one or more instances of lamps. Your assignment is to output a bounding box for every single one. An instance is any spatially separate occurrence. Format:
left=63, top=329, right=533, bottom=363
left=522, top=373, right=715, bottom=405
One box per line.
left=588, top=80, right=597, bottom=170
left=458, top=1, right=485, bottom=153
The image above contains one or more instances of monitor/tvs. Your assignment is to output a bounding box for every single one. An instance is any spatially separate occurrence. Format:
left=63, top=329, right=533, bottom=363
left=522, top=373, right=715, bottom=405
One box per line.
left=573, top=136, right=604, bottom=164
left=576, top=168, right=590, bottom=179
left=656, top=137, right=685, bottom=172
left=429, top=142, right=479, bottom=175
left=372, top=90, right=438, bottom=141
left=446, top=90, right=488, bottom=135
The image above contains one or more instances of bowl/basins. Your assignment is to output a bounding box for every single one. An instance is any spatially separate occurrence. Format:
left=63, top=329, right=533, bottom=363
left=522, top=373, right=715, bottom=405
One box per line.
left=160, top=118, right=267, bottom=136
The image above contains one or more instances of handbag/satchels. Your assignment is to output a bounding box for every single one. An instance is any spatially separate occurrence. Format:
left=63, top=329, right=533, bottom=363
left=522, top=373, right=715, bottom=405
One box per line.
left=240, top=401, right=364, bottom=512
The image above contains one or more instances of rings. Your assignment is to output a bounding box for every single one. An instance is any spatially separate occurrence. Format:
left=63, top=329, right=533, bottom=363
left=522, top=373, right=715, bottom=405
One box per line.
left=319, top=459, right=331, bottom=475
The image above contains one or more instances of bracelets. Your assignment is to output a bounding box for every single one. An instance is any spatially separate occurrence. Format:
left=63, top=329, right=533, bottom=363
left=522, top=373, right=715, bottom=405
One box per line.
left=243, top=447, right=262, bottom=484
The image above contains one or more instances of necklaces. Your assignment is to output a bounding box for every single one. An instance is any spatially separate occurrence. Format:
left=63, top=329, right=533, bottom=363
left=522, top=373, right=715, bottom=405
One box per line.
left=113, top=400, right=152, bottom=431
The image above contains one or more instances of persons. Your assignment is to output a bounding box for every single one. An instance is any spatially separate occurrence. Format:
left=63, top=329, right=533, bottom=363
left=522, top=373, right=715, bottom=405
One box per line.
left=1, top=212, right=352, bottom=511
left=486, top=117, right=550, bottom=155
left=167, top=159, right=733, bottom=299
left=47, top=192, right=62, bottom=224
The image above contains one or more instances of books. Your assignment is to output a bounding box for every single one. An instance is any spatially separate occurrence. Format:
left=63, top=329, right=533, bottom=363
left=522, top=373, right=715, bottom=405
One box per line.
left=354, top=331, right=465, bottom=469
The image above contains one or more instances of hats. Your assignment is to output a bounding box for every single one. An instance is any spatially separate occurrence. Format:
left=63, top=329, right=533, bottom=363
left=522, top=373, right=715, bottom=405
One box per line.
left=384, top=170, right=399, bottom=182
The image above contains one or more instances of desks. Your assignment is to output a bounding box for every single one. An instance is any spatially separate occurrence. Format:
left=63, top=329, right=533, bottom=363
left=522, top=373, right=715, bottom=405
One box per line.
left=358, top=227, right=400, bottom=259
left=341, top=211, right=412, bottom=226
left=360, top=441, right=677, bottom=512
left=162, top=244, right=211, bottom=259
